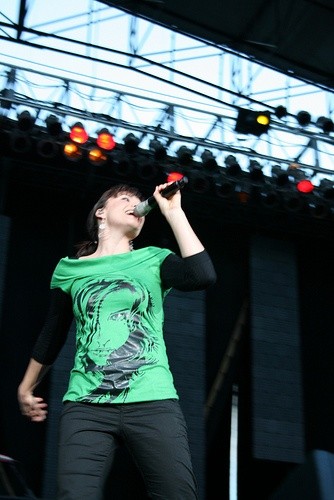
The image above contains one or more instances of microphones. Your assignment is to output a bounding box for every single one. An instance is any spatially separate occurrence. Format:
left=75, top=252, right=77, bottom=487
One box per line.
left=134, top=176, right=188, bottom=217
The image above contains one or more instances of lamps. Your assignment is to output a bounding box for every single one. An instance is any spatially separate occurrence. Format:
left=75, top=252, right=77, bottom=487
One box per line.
left=17, top=105, right=334, bottom=199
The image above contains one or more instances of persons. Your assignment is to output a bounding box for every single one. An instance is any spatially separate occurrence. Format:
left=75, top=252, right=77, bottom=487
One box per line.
left=18, top=179, right=219, bottom=500
